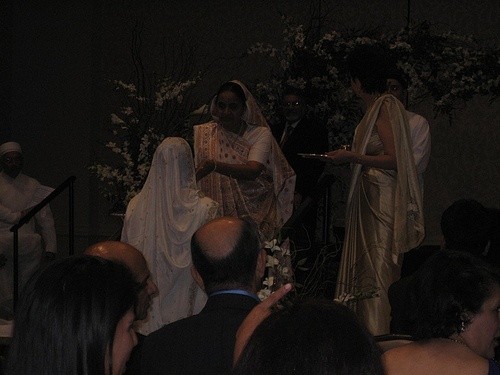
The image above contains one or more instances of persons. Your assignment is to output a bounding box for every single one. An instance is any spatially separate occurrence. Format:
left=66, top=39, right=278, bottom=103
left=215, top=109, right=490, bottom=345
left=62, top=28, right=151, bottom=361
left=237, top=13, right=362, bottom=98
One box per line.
left=134, top=217, right=265, bottom=375
left=193, top=78, right=296, bottom=296
left=379, top=250, right=500, bottom=375
left=390, top=196, right=499, bottom=336
left=265, top=85, right=328, bottom=275
left=120, top=135, right=218, bottom=336
left=0, top=254, right=137, bottom=375
left=0, top=141, right=57, bottom=325
left=230, top=298, right=385, bottom=375
left=382, top=68, right=410, bottom=108
left=80, top=241, right=159, bottom=375
left=327, top=47, right=426, bottom=338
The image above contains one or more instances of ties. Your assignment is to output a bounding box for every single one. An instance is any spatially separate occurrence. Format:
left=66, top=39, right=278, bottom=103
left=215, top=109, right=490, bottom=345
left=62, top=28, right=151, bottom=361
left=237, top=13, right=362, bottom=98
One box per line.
left=280, top=126, right=292, bottom=149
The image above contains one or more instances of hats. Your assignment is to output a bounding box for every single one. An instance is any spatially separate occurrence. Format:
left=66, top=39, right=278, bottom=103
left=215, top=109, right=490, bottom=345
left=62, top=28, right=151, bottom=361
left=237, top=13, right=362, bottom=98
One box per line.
left=0, top=142, right=22, bottom=157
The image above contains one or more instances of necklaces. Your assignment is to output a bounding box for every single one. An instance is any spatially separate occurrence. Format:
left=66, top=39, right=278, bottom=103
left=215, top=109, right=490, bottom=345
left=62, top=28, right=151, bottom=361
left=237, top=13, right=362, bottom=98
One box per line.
left=221, top=119, right=244, bottom=134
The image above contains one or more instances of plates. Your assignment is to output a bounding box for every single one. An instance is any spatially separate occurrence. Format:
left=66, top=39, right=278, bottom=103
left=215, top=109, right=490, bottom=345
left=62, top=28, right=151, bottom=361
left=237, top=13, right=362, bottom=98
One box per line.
left=297, top=154, right=329, bottom=158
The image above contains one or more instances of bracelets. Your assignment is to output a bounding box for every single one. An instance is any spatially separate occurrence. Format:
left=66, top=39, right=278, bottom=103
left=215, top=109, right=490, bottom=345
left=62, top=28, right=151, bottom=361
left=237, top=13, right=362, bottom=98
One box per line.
left=213, top=159, right=221, bottom=175
